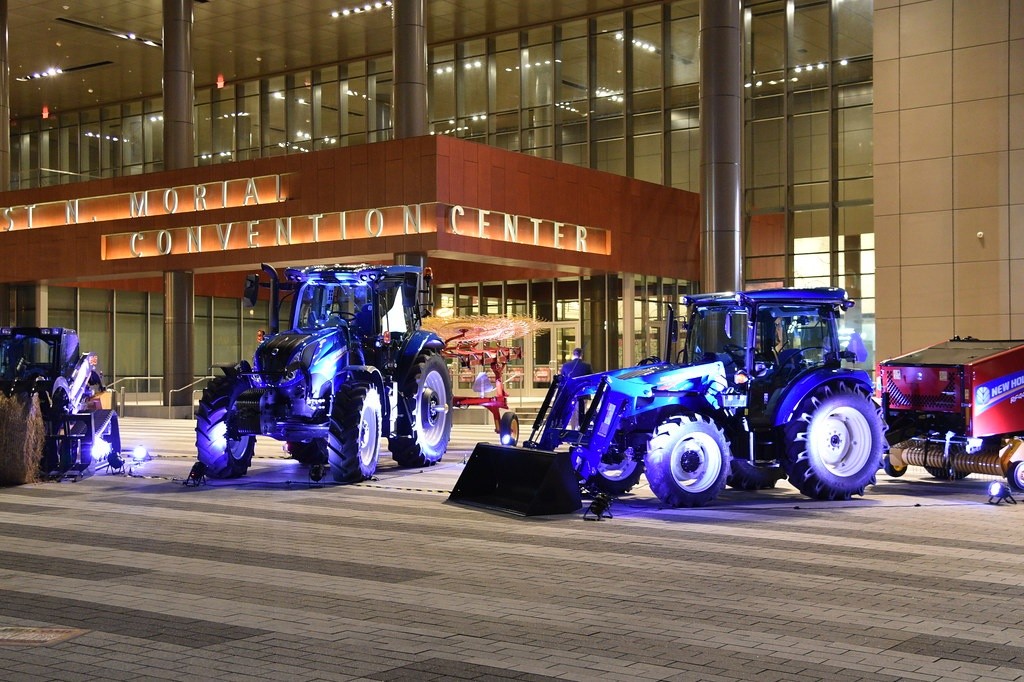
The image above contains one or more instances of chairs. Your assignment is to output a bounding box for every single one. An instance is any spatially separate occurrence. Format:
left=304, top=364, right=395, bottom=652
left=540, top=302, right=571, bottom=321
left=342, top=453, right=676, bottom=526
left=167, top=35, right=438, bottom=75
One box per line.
left=22, top=364, right=51, bottom=381
left=353, top=303, right=383, bottom=337
left=757, top=349, right=801, bottom=385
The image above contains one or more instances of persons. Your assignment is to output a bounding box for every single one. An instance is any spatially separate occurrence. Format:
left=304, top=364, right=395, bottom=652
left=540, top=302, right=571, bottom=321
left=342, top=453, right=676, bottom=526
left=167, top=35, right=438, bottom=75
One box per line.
left=560, top=348, right=595, bottom=430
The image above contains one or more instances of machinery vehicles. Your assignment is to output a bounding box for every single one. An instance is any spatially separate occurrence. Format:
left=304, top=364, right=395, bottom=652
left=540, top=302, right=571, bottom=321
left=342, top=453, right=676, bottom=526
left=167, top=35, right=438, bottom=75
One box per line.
left=0, top=327, right=122, bottom=487
left=195, top=262, right=454, bottom=483
left=442, top=287, right=890, bottom=522
